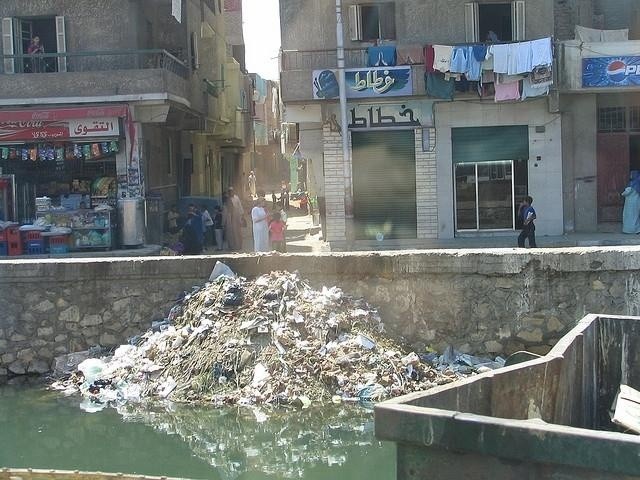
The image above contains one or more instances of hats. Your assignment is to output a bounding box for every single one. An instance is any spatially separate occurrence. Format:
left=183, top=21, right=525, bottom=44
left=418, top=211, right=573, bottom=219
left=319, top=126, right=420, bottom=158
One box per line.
left=170, top=196, right=222, bottom=244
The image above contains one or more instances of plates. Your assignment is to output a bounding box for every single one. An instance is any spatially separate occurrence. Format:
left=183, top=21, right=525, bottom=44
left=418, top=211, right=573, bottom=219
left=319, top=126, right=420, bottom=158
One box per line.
left=35, top=209, right=118, bottom=248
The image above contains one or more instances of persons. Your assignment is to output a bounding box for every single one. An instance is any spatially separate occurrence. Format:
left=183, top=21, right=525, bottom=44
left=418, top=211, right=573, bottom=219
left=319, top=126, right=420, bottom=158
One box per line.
left=165, top=185, right=291, bottom=253
left=517, top=195, right=538, bottom=248
left=620, top=168, right=640, bottom=234
left=248, top=171, right=257, bottom=197
left=27, top=33, right=47, bottom=72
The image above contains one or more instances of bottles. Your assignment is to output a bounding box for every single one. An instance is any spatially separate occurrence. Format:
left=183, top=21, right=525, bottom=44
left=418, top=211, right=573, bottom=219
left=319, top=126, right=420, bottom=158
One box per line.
left=249, top=171, right=254, bottom=175
